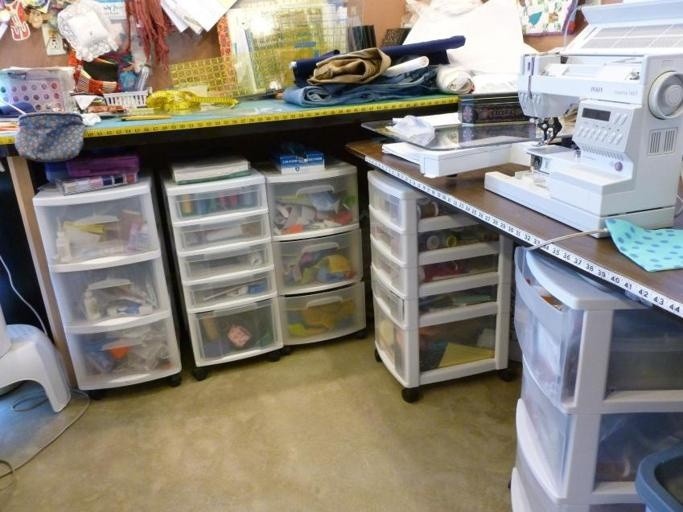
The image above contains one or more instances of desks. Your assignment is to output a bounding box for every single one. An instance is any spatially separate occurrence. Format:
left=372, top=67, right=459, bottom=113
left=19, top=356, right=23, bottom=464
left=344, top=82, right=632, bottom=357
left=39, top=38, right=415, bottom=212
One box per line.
left=0, top=91, right=465, bottom=390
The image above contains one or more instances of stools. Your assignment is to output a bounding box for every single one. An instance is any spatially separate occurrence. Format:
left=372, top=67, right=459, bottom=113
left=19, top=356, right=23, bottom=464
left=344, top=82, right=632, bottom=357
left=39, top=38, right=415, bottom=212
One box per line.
left=0, top=324, right=74, bottom=414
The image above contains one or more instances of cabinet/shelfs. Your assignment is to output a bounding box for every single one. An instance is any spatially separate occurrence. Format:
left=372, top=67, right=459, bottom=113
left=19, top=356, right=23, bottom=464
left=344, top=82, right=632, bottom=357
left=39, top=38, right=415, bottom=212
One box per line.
left=367, top=169, right=515, bottom=406
left=509, top=239, right=682, bottom=512
left=161, top=166, right=284, bottom=381
left=33, top=170, right=183, bottom=392
left=262, top=161, right=369, bottom=353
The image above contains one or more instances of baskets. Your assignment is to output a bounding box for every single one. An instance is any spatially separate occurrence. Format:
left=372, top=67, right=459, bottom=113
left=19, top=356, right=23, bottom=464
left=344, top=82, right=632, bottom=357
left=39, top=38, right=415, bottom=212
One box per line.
left=103, top=90, right=149, bottom=109
left=1, top=68, right=77, bottom=116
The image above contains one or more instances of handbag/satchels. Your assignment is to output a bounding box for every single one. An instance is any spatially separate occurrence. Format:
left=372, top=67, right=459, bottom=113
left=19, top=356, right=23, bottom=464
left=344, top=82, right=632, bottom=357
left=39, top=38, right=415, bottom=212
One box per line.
left=3, top=101, right=85, bottom=164
